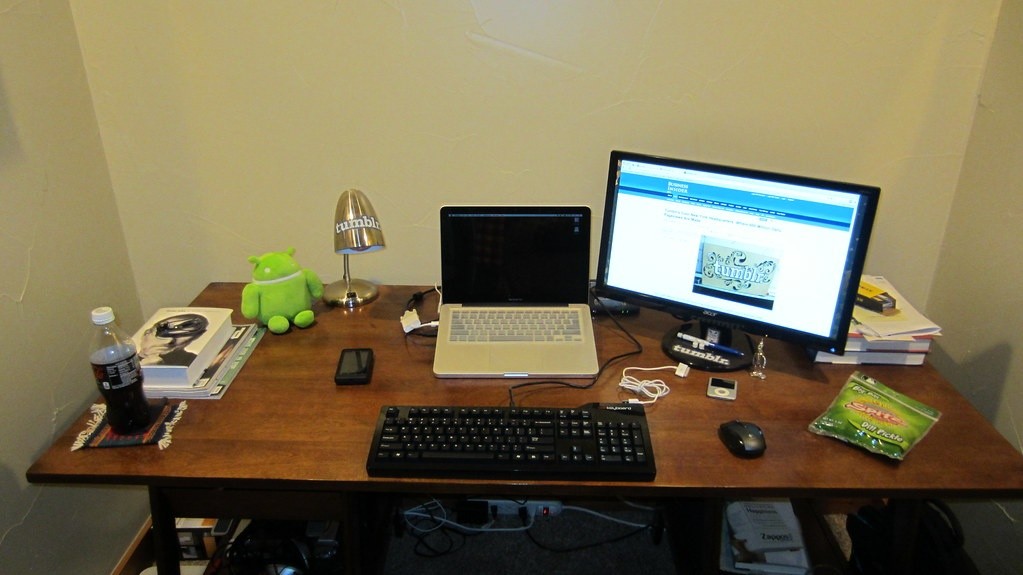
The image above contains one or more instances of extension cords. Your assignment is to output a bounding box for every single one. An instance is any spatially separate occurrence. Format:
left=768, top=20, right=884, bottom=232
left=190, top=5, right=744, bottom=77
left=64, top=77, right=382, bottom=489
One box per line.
left=468, top=499, right=562, bottom=516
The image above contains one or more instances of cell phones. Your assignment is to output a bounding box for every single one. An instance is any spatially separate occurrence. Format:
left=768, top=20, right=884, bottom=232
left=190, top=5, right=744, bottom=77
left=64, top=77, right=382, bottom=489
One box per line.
left=334, top=347, right=373, bottom=385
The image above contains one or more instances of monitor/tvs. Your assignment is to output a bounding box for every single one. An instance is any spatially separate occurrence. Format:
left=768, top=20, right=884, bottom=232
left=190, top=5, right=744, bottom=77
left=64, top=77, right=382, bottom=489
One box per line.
left=594, top=149, right=881, bottom=372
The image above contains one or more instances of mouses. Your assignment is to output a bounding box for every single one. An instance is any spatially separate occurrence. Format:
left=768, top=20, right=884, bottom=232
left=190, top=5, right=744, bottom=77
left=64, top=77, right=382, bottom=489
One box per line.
left=719, top=419, right=767, bottom=460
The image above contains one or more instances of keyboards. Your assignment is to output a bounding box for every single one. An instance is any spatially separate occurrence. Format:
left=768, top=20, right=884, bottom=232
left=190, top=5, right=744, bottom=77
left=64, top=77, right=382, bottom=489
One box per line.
left=366, top=403, right=657, bottom=485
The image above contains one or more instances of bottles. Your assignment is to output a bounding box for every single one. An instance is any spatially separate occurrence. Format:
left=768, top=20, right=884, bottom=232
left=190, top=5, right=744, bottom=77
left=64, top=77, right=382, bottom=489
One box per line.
left=88, top=306, right=154, bottom=435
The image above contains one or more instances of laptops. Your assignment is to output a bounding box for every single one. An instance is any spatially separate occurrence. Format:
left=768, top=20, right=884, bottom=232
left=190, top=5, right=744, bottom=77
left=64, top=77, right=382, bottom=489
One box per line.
left=433, top=205, right=599, bottom=379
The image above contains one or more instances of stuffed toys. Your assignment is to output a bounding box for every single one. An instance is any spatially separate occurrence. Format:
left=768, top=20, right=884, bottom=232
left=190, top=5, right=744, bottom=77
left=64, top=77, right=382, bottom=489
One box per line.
left=241, top=248, right=324, bottom=334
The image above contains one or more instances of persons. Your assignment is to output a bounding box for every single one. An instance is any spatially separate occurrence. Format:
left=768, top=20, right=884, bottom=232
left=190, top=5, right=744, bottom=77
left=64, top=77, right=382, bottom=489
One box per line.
left=137, top=314, right=208, bottom=366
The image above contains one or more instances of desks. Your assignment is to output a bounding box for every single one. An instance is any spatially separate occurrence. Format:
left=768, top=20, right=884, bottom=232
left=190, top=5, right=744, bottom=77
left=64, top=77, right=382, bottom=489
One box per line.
left=25, top=280, right=1023, bottom=575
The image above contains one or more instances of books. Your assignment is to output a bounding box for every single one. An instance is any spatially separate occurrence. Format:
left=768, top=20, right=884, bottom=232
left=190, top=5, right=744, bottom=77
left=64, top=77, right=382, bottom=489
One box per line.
left=809, top=275, right=932, bottom=364
left=128, top=306, right=266, bottom=399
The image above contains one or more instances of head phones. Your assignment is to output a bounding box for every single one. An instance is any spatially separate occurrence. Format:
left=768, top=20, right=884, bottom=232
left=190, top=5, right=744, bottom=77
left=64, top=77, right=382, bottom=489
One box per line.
left=228, top=535, right=312, bottom=575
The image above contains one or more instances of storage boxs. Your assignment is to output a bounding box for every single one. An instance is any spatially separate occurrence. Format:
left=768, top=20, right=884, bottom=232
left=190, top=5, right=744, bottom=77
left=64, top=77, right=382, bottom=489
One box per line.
left=334, top=348, right=374, bottom=385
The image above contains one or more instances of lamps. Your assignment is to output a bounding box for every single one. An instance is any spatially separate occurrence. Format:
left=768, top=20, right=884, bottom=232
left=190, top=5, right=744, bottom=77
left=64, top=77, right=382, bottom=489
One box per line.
left=322, top=188, right=386, bottom=307
